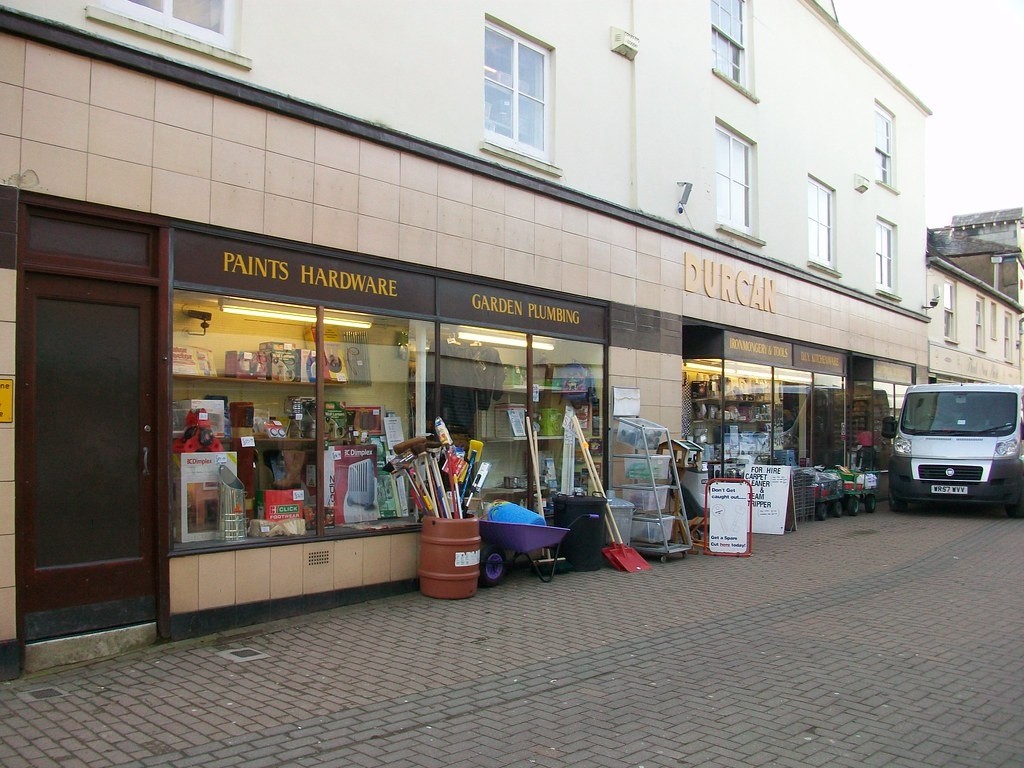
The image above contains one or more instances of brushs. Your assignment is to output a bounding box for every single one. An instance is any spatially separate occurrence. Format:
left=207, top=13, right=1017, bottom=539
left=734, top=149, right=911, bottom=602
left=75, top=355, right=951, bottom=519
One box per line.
left=382, top=418, right=492, bottom=518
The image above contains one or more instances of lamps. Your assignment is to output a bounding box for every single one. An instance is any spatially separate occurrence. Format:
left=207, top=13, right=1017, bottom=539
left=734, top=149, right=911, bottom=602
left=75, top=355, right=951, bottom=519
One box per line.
left=921, top=298, right=938, bottom=310
left=217, top=298, right=374, bottom=329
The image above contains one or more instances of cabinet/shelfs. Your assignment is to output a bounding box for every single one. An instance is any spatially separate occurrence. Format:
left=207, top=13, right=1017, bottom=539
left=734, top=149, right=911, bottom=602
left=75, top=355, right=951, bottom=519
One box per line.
left=173, top=374, right=349, bottom=442
left=690, top=371, right=773, bottom=477
left=482, top=386, right=565, bottom=496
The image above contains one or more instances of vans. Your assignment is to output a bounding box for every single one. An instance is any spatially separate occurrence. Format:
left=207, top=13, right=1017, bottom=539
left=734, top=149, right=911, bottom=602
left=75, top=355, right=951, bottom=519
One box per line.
left=880, top=383, right=1024, bottom=520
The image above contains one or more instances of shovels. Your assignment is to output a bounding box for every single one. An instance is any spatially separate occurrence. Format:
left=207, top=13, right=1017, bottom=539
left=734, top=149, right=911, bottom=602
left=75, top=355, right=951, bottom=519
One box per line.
left=569, top=415, right=651, bottom=572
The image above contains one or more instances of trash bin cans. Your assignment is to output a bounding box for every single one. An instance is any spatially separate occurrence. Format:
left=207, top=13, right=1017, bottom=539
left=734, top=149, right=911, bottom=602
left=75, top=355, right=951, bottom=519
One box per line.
left=599, top=488, right=636, bottom=547
left=552, top=491, right=607, bottom=571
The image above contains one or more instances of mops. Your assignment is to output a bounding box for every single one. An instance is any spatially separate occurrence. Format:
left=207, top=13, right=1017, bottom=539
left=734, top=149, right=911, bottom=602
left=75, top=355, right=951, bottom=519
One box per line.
left=434, top=416, right=492, bottom=519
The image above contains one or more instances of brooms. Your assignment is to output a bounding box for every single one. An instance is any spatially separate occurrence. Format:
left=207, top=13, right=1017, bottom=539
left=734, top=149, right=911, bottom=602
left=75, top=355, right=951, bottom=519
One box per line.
left=381, top=436, right=454, bottom=519
left=525, top=416, right=574, bottom=576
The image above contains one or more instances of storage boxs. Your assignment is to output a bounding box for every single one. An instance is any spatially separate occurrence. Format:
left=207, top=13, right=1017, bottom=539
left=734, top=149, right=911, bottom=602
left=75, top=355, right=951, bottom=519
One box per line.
left=621, top=487, right=668, bottom=511
left=615, top=419, right=663, bottom=449
left=295, top=349, right=316, bottom=382
left=304, top=323, right=348, bottom=384
left=623, top=455, right=670, bottom=479
left=302, top=444, right=378, bottom=525
left=536, top=408, right=564, bottom=436
left=632, top=514, right=676, bottom=543
left=169, top=452, right=237, bottom=543
left=502, top=364, right=526, bottom=389
left=495, top=403, right=526, bottom=437
left=259, top=342, right=296, bottom=382
left=225, top=351, right=266, bottom=380
left=255, top=489, right=303, bottom=520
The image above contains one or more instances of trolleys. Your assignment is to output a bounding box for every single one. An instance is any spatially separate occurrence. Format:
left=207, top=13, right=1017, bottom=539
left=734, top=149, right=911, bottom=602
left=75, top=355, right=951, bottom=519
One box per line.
left=815, top=469, right=844, bottom=522
left=839, top=468, right=888, bottom=517
left=478, top=510, right=600, bottom=589
